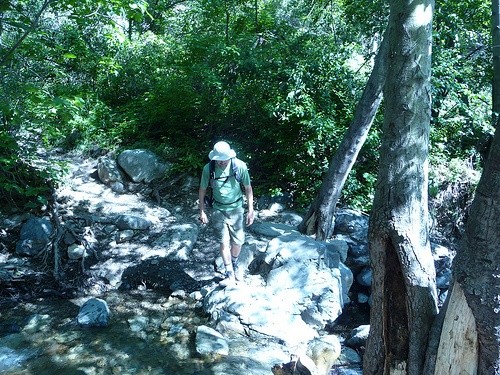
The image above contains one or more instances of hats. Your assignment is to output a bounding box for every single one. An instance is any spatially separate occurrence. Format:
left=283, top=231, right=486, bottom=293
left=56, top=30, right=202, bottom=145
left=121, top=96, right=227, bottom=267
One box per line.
left=207, top=141, right=237, bottom=161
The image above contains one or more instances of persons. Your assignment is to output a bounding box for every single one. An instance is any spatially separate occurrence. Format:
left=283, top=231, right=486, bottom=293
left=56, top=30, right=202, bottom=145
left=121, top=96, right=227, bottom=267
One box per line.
left=199, top=141, right=254, bottom=287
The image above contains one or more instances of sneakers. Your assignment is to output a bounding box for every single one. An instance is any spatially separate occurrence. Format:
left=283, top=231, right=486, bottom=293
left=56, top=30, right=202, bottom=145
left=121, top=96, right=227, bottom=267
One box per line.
left=232, top=261, right=244, bottom=281
left=219, top=270, right=235, bottom=286
